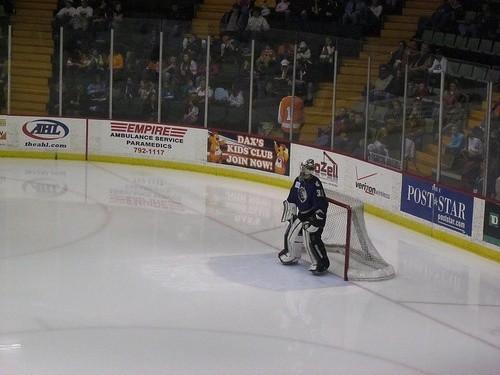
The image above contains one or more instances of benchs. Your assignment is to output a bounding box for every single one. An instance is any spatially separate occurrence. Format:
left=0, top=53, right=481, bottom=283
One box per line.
left=4, top=0, right=500, bottom=180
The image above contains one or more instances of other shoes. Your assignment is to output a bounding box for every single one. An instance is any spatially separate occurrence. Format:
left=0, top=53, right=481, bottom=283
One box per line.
left=279, top=249, right=300, bottom=264
left=309, top=258, right=330, bottom=274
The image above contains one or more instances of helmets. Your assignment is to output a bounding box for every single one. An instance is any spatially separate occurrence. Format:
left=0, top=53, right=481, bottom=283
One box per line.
left=300, top=159, right=315, bottom=174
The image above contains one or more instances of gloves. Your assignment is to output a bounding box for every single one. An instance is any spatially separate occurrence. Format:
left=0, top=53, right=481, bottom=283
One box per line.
left=306, top=225, right=319, bottom=233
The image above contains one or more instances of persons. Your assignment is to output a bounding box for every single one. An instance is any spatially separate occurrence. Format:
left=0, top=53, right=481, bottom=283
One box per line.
left=49, top=0, right=500, bottom=199
left=277, top=159, right=330, bottom=274
